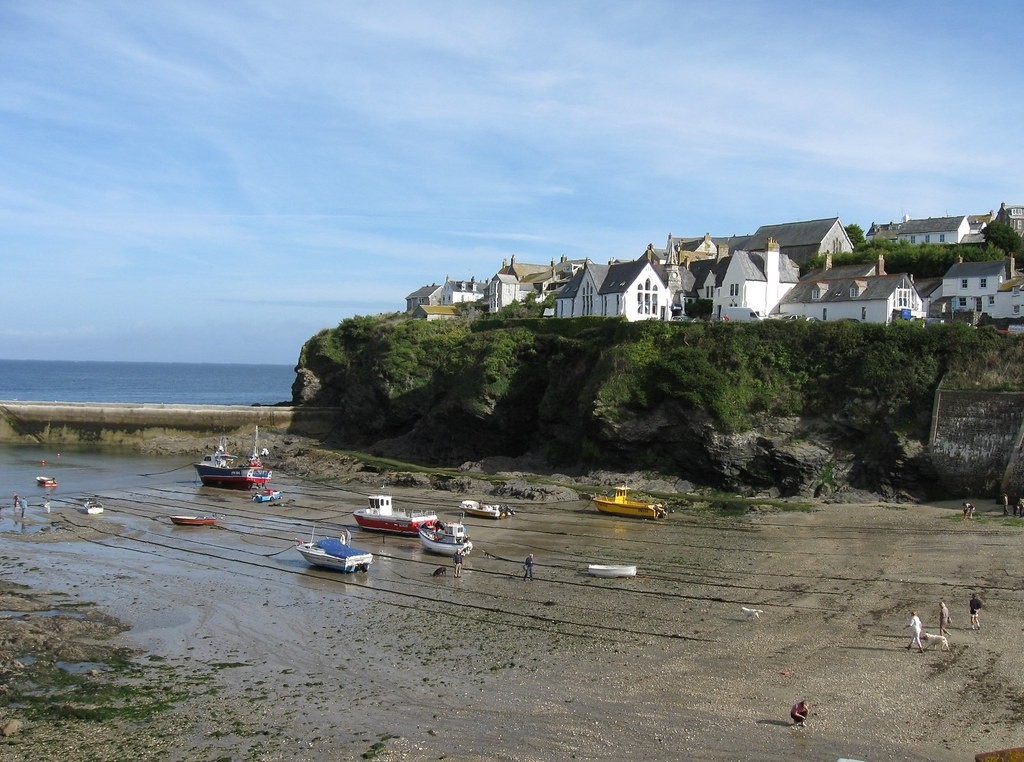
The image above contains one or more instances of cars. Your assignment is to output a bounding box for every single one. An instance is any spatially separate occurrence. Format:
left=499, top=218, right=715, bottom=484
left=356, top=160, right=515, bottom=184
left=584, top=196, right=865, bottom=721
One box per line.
left=672, top=316, right=696, bottom=322
left=782, top=314, right=861, bottom=323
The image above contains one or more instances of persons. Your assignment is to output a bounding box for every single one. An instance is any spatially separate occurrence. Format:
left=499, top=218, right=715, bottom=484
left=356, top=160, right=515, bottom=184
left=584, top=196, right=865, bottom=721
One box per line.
left=969, top=594, right=982, bottom=629
left=44, top=500, right=50, bottom=514
left=345, top=528, right=352, bottom=548
left=453, top=549, right=463, bottom=578
left=21, top=496, right=27, bottom=519
left=963, top=501, right=976, bottom=511
left=1012, top=492, right=1020, bottom=515
left=903, top=611, right=924, bottom=652
left=523, top=553, right=534, bottom=580
left=339, top=531, right=345, bottom=545
left=12, top=491, right=19, bottom=507
left=1003, top=493, right=1010, bottom=515
left=790, top=700, right=809, bottom=726
left=938, top=601, right=953, bottom=636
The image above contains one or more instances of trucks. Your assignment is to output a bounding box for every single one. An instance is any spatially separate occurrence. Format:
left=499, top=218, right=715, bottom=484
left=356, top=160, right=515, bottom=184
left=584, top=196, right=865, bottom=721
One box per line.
left=728, top=308, right=762, bottom=321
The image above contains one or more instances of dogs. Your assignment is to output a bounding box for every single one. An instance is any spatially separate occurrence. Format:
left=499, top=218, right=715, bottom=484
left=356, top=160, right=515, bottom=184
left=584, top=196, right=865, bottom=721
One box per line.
left=922, top=633, right=949, bottom=651
left=433, top=567, right=447, bottom=577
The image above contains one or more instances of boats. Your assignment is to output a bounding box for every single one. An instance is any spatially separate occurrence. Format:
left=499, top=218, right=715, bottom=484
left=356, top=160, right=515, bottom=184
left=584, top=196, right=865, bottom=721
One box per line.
left=83, top=497, right=103, bottom=514
left=588, top=564, right=637, bottom=579
left=353, top=494, right=437, bottom=537
left=418, top=523, right=473, bottom=557
left=593, top=484, right=663, bottom=520
left=295, top=538, right=373, bottom=574
left=251, top=489, right=283, bottom=502
left=192, top=426, right=273, bottom=490
left=36, top=476, right=57, bottom=487
left=459, top=500, right=515, bottom=519
left=170, top=515, right=216, bottom=525
